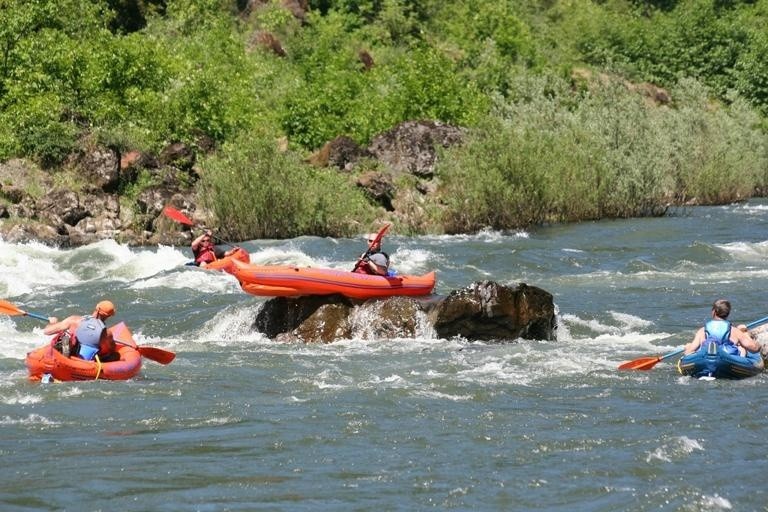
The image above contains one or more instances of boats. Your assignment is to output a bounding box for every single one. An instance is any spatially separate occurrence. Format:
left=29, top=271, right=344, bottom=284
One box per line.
left=200, top=245, right=251, bottom=270
left=25, top=319, right=143, bottom=381
left=677, top=338, right=763, bottom=375
left=229, top=255, right=437, bottom=303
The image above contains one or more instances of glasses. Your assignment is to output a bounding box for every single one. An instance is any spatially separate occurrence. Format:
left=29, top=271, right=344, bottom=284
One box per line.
left=201, top=239, right=210, bottom=242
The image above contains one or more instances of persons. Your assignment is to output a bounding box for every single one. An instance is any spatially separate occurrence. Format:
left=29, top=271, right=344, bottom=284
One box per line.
left=190, top=230, right=242, bottom=266
left=44, top=299, right=120, bottom=360
left=684, top=297, right=760, bottom=358
left=355, top=233, right=389, bottom=276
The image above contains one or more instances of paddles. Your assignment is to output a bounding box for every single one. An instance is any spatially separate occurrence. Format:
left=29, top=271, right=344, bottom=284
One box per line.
left=352, top=224, right=390, bottom=272
left=163, top=207, right=239, bottom=249
left=0, top=301, right=176, bottom=365
left=618, top=317, right=767, bottom=370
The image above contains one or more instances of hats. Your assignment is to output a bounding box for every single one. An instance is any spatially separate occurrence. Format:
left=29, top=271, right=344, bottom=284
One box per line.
left=95, top=300, right=115, bottom=317
